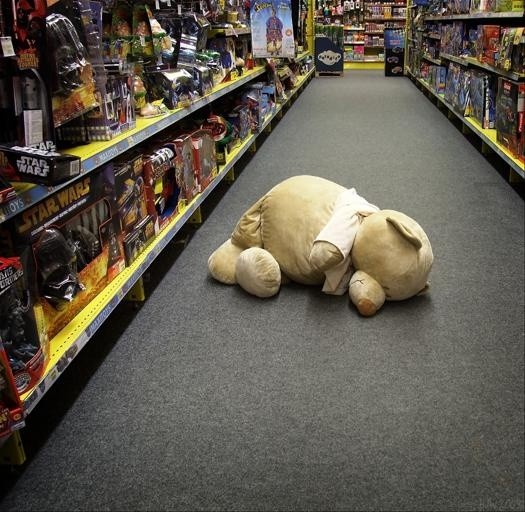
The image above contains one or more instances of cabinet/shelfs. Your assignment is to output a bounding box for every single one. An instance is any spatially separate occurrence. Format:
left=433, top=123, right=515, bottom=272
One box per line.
left=315, top=24, right=344, bottom=77
left=315, top=0, right=408, bottom=63
left=384, top=28, right=405, bottom=77
left=403, top=0, right=525, bottom=182
left=0, top=0, right=316, bottom=464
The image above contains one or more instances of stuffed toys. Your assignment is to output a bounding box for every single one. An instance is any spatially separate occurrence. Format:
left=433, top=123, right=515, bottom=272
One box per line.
left=207, top=174, right=434, bottom=317
